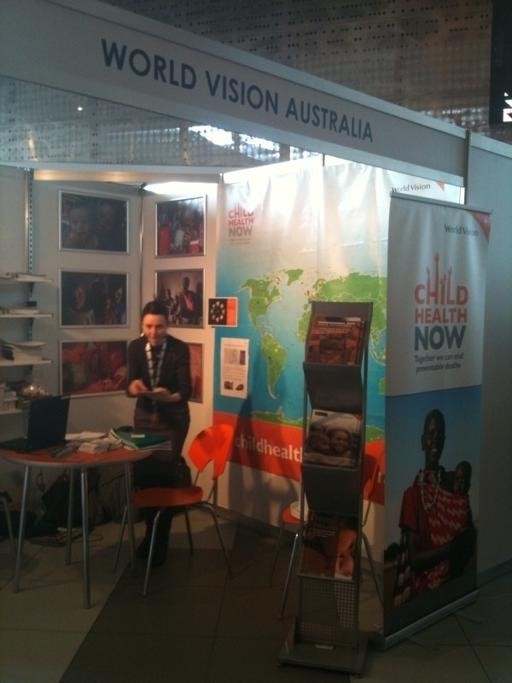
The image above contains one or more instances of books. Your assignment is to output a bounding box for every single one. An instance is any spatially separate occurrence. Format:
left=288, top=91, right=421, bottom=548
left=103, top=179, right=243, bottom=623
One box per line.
left=293, top=311, right=367, bottom=584
left=1, top=268, right=49, bottom=405
left=64, top=423, right=173, bottom=457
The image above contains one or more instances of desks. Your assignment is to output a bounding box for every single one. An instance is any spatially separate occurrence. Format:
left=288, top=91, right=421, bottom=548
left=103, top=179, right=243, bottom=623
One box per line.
left=0, top=449, right=153, bottom=609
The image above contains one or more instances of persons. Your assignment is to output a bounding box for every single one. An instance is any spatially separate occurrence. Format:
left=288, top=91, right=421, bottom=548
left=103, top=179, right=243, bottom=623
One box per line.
left=61, top=341, right=126, bottom=396
left=392, top=403, right=478, bottom=632
left=61, top=274, right=127, bottom=325
left=154, top=200, right=204, bottom=257
left=69, top=199, right=128, bottom=249
left=416, top=458, right=474, bottom=573
left=158, top=277, right=202, bottom=326
left=60, top=200, right=98, bottom=249
left=124, top=298, right=194, bottom=571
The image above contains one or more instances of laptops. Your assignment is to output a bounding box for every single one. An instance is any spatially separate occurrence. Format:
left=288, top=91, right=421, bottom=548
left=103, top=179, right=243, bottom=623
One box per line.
left=0, top=393, right=70, bottom=451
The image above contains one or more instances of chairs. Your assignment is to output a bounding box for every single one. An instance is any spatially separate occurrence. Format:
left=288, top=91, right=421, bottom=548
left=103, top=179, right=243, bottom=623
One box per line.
left=267, top=439, right=387, bottom=621
left=0, top=494, right=16, bottom=559
left=111, top=424, right=237, bottom=597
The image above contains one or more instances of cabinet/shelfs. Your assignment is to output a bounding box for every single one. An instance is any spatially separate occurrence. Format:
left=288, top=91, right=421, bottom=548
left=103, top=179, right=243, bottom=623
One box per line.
left=0, top=272, right=54, bottom=416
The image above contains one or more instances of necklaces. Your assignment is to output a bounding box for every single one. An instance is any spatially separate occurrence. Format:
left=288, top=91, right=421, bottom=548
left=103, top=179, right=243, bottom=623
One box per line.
left=419, top=469, right=443, bottom=514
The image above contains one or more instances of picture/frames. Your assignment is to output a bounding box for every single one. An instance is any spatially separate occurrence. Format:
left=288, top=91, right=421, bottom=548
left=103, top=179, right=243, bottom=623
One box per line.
left=57, top=190, right=130, bottom=254
left=206, top=297, right=239, bottom=328
left=59, top=268, right=129, bottom=329
left=154, top=193, right=207, bottom=256
left=154, top=267, right=206, bottom=330
left=180, top=341, right=206, bottom=407
left=57, top=338, right=132, bottom=400
